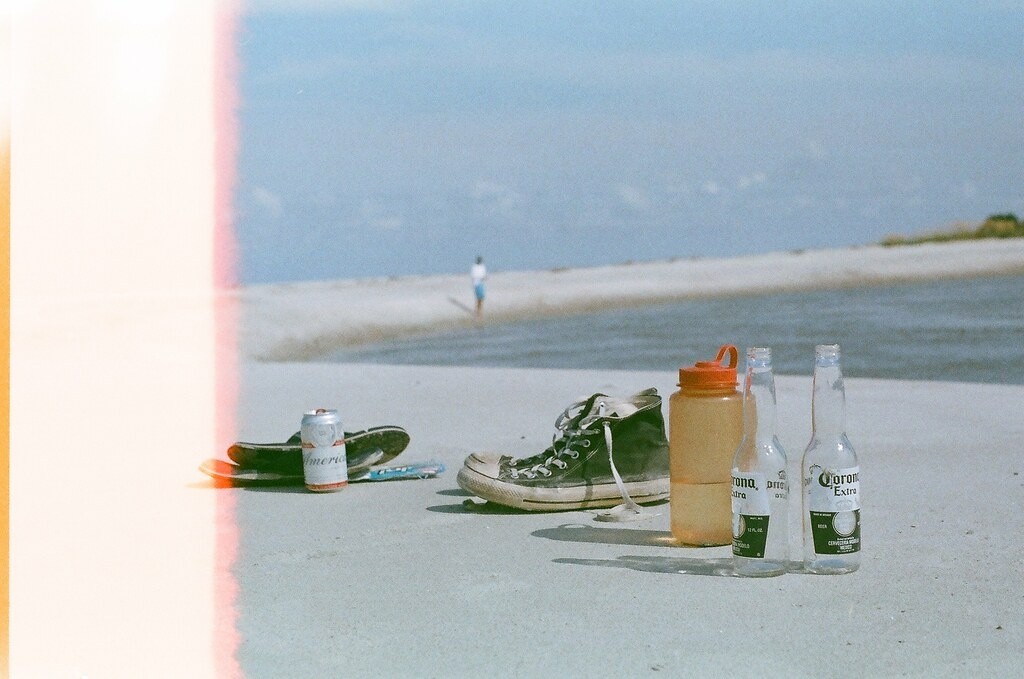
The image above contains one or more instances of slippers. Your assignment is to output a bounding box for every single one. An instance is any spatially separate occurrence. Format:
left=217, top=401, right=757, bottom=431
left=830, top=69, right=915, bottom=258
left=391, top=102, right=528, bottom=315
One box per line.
left=227, top=425, right=410, bottom=473
left=198, top=447, right=383, bottom=485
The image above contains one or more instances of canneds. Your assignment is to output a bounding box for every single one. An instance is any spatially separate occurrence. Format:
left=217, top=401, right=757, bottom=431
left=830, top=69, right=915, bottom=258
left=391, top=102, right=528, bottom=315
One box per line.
left=301, top=409, right=348, bottom=491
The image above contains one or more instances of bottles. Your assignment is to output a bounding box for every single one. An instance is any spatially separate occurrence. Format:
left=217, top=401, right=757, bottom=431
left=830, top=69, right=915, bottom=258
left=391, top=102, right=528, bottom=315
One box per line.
left=732, top=346, right=788, bottom=578
left=803, top=344, right=861, bottom=576
left=668, top=345, right=744, bottom=546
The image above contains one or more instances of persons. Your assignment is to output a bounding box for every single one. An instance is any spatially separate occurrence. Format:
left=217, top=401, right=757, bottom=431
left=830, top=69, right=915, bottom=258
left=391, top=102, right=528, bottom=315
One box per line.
left=471, top=256, right=487, bottom=315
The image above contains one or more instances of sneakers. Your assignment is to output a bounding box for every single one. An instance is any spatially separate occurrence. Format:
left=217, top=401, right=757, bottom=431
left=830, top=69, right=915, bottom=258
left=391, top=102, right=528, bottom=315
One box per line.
left=457, top=386, right=671, bottom=524
